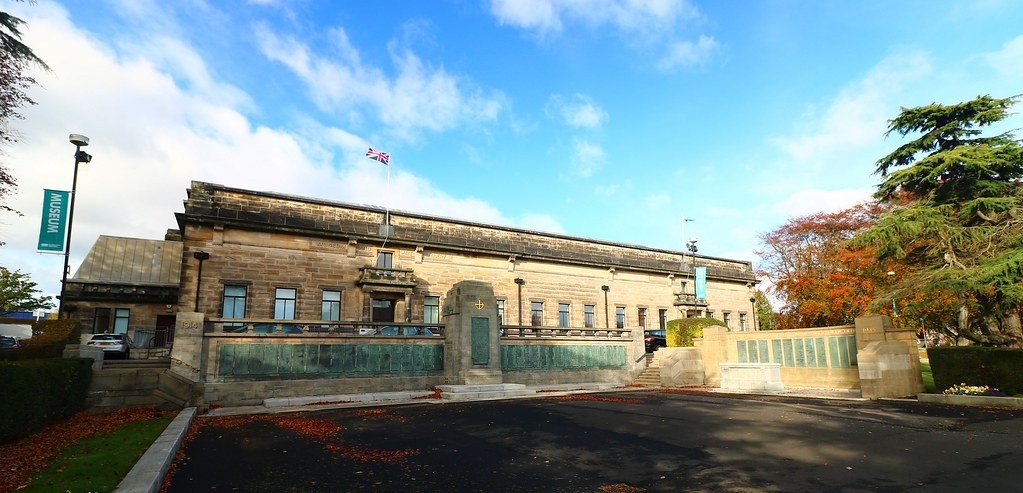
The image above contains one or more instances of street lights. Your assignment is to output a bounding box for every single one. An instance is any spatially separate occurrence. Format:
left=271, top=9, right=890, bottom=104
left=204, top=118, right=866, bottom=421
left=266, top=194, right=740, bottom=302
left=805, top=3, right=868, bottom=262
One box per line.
left=514, top=277, right=524, bottom=337
left=56, top=135, right=92, bottom=318
left=602, top=285, right=609, bottom=333
left=686, top=236, right=699, bottom=318
left=919, top=318, right=927, bottom=347
left=887, top=271, right=895, bottom=318
left=750, top=297, right=757, bottom=330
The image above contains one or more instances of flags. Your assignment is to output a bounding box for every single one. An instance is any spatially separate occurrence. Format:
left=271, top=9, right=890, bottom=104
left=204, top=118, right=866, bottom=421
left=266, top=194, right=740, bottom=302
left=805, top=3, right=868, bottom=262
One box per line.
left=365, top=147, right=390, bottom=165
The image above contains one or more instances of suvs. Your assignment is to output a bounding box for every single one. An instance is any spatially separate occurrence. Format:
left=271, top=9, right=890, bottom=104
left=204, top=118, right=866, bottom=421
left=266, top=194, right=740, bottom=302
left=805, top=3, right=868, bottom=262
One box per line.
left=644, top=330, right=668, bottom=352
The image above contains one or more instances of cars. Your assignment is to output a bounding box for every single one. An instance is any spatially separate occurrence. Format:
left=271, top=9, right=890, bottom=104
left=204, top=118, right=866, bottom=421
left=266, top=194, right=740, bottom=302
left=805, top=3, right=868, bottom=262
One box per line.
left=232, top=323, right=302, bottom=334
left=365, top=327, right=436, bottom=338
left=87, top=334, right=131, bottom=359
left=0, top=336, right=22, bottom=348
